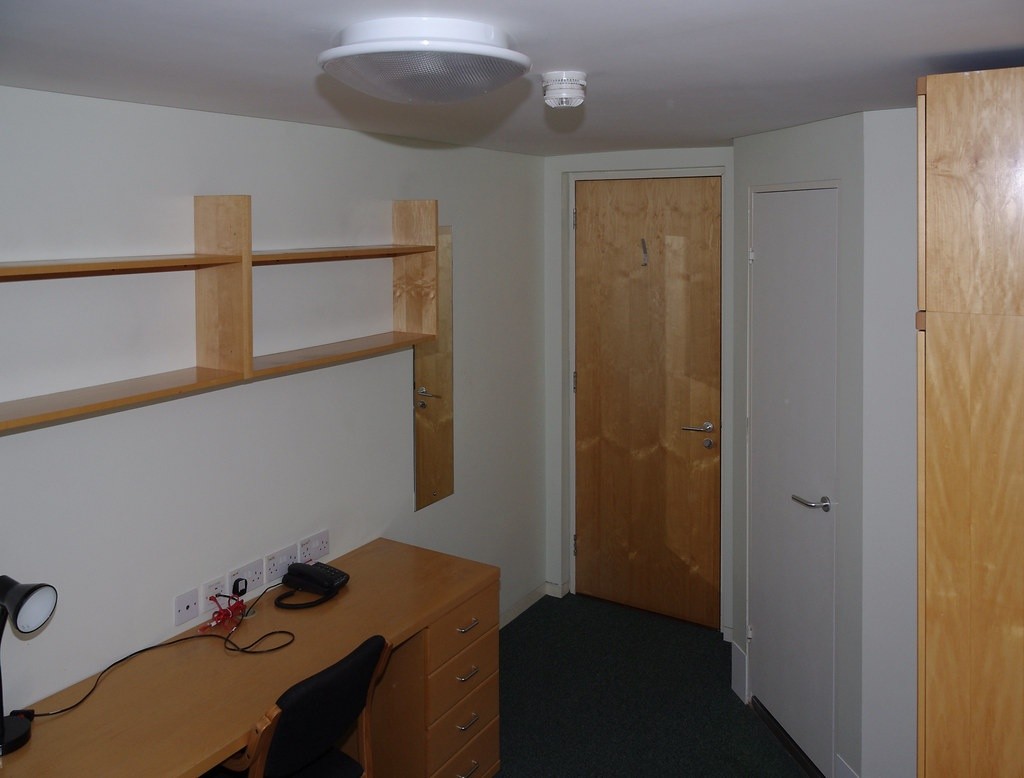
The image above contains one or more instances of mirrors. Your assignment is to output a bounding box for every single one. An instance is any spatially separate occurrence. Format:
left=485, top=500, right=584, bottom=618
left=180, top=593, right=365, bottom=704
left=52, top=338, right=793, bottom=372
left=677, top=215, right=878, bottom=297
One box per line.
left=414, top=225, right=454, bottom=511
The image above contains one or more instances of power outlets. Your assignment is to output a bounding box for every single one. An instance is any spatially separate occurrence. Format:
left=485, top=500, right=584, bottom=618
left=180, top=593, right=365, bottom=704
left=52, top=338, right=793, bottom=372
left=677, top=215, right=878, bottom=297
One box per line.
left=202, top=577, right=225, bottom=610
left=229, top=559, right=263, bottom=598
left=264, top=542, right=298, bottom=583
left=299, top=530, right=330, bottom=564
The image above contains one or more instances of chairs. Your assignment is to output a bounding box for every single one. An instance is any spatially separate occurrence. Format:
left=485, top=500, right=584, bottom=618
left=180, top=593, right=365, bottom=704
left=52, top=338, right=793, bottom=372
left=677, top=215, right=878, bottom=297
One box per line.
left=201, top=635, right=394, bottom=778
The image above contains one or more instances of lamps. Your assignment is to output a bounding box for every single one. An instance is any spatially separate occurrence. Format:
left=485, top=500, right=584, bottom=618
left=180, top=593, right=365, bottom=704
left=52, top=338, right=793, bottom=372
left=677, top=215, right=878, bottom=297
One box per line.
left=317, top=18, right=530, bottom=104
left=0, top=575, right=57, bottom=757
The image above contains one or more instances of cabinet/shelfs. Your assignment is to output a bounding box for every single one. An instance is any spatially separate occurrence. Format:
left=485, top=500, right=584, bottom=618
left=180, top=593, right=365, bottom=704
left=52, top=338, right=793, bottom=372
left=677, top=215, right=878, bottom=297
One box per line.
left=0, top=196, right=438, bottom=432
left=918, top=67, right=1022, bottom=778
left=335, top=580, right=501, bottom=778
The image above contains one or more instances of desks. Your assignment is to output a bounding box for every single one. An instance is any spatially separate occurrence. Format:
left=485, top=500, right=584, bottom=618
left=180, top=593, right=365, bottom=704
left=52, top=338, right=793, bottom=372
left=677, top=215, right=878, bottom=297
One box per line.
left=0, top=537, right=501, bottom=778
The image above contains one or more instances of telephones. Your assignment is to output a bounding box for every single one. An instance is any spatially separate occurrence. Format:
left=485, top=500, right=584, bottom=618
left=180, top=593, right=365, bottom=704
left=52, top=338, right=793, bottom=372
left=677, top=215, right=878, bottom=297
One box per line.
left=281, top=558, right=350, bottom=596
